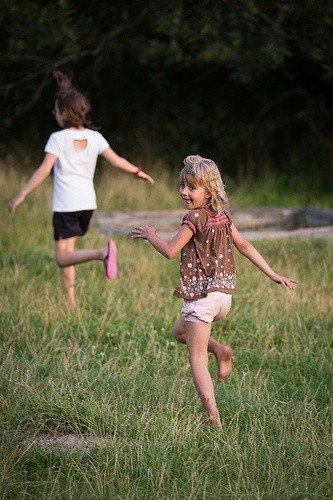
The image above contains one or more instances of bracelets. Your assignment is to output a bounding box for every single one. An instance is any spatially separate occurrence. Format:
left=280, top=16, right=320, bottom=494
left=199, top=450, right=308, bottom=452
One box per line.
left=133, top=167, right=141, bottom=175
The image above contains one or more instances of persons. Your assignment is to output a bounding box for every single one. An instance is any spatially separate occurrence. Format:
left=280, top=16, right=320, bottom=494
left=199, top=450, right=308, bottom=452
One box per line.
left=9, top=73, right=154, bottom=309
left=131, top=156, right=300, bottom=428
